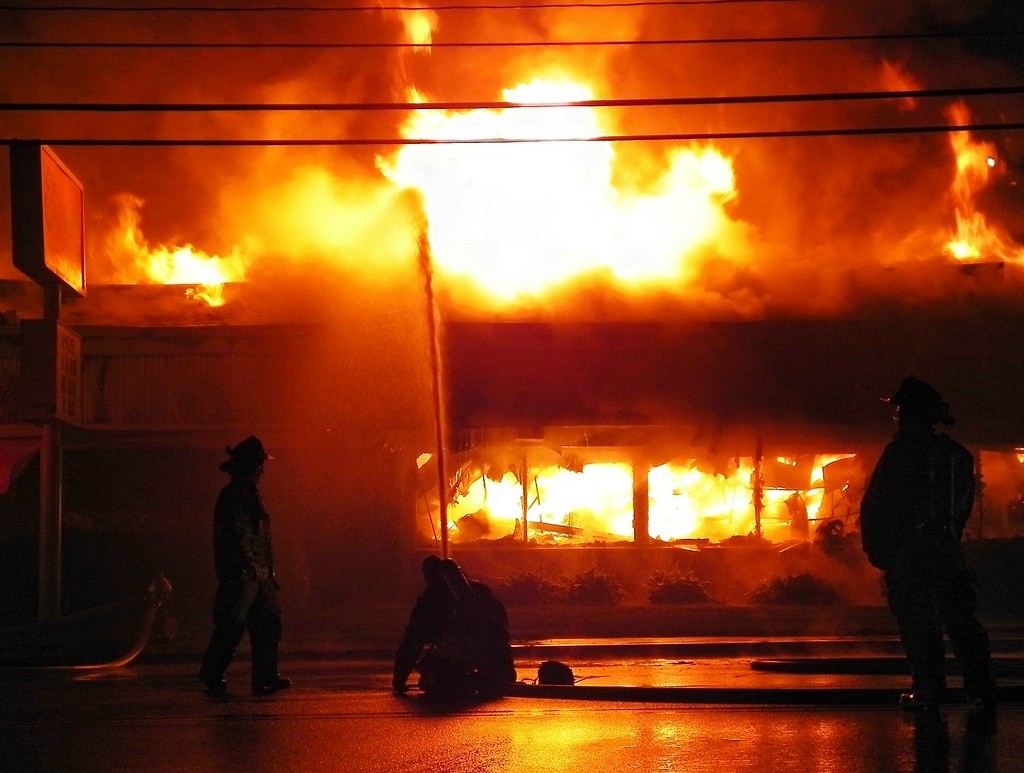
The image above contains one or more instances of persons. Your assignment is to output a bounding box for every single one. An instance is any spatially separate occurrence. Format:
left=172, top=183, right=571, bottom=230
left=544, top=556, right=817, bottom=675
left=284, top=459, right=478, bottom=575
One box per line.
left=859, top=378, right=999, bottom=773
left=391, top=556, right=517, bottom=700
left=201, top=433, right=293, bottom=698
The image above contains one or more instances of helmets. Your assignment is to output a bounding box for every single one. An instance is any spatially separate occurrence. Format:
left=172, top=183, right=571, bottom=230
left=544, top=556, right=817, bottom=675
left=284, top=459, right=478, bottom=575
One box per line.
left=880, top=376, right=956, bottom=427
left=220, top=436, right=274, bottom=474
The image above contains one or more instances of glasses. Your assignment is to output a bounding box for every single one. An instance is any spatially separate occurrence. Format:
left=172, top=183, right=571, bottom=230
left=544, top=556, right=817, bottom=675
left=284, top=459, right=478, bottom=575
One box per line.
left=890, top=409, right=903, bottom=421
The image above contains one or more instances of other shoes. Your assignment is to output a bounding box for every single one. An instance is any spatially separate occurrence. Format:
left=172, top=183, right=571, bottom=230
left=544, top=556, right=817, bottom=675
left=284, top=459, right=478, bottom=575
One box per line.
left=252, top=672, right=292, bottom=692
left=899, top=693, right=943, bottom=710
left=961, top=695, right=995, bottom=711
left=200, top=676, right=226, bottom=697
